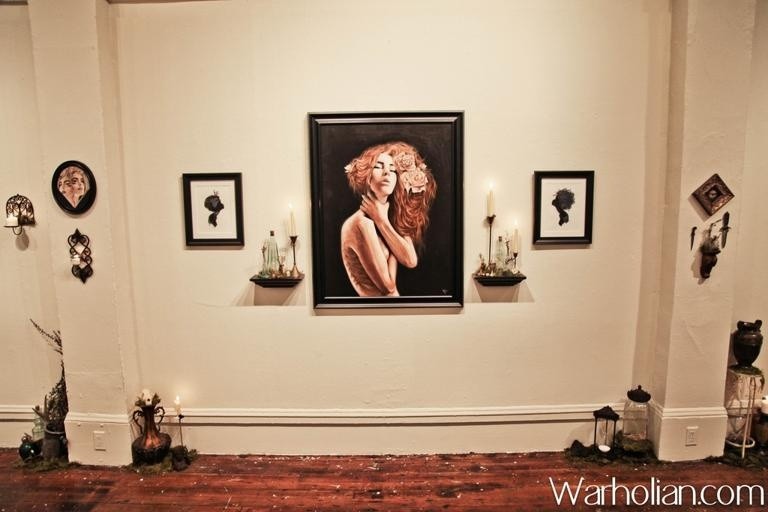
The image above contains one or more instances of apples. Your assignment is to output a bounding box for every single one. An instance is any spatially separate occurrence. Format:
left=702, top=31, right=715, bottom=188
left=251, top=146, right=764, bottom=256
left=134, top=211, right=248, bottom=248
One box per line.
left=6, top=213, right=18, bottom=226
left=174, top=394, right=182, bottom=414
left=487, top=178, right=494, bottom=215
left=511, top=220, right=518, bottom=255
left=71, top=253, right=80, bottom=264
left=287, top=203, right=296, bottom=236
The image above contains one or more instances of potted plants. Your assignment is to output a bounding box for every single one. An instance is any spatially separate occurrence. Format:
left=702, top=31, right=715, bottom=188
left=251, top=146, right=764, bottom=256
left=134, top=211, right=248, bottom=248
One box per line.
left=531, top=169, right=595, bottom=249
left=306, top=108, right=467, bottom=312
left=181, top=169, right=244, bottom=250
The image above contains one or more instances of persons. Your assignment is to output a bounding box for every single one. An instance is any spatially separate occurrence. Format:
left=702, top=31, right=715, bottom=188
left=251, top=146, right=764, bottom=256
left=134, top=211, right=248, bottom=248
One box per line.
left=59, top=167, right=86, bottom=206
left=338, top=150, right=418, bottom=296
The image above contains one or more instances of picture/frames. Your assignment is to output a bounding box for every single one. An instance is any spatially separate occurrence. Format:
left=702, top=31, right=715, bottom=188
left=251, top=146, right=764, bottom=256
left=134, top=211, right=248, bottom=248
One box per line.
left=723, top=364, right=764, bottom=460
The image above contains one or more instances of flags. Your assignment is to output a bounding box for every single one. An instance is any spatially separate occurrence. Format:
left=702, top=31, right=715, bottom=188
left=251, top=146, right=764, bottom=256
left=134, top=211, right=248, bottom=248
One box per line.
left=131, top=395, right=172, bottom=465
left=19, top=437, right=40, bottom=465
left=42, top=426, right=65, bottom=460
left=729, top=318, right=764, bottom=369
